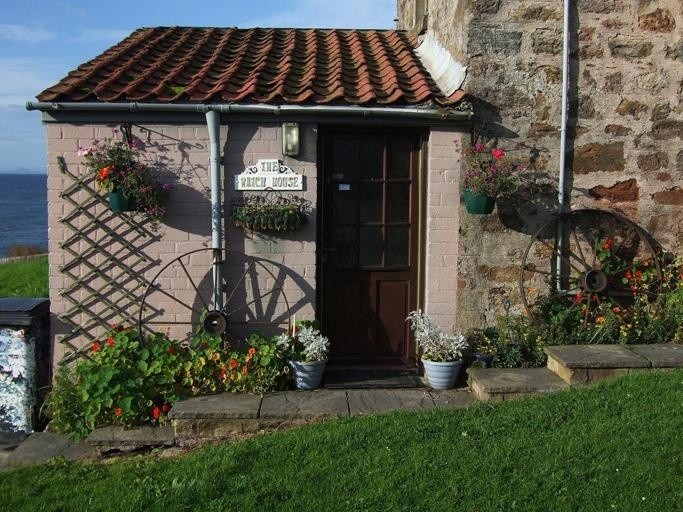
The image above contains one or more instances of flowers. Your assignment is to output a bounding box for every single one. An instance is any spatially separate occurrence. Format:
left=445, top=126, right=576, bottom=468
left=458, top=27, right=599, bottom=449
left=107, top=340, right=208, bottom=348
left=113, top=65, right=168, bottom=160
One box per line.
left=276, top=325, right=329, bottom=361
left=403, top=309, right=469, bottom=359
left=76, top=128, right=167, bottom=219
left=453, top=139, right=521, bottom=196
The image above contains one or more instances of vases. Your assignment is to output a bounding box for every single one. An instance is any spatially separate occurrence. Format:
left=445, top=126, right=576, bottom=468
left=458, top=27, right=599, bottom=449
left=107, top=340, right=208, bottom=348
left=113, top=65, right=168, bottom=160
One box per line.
left=461, top=189, right=495, bottom=216
left=288, top=360, right=323, bottom=389
left=106, top=180, right=141, bottom=212
left=420, top=355, right=461, bottom=390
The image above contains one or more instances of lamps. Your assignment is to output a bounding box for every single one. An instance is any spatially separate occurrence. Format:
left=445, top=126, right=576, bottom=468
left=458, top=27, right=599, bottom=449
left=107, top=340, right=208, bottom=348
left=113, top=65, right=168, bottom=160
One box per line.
left=281, top=122, right=299, bottom=156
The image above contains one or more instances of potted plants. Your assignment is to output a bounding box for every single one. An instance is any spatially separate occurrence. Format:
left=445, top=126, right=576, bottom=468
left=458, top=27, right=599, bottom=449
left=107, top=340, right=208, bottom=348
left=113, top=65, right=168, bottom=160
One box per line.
left=228, top=202, right=310, bottom=233
